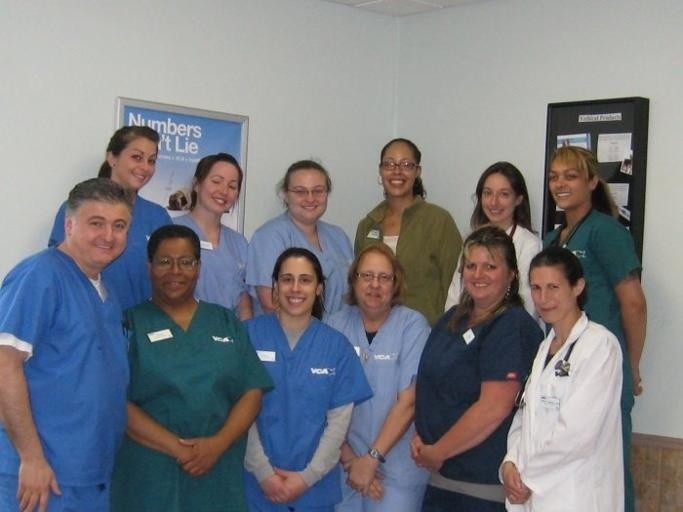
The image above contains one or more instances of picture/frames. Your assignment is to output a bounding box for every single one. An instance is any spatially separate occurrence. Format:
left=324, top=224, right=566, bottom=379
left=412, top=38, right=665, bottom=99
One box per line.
left=114, top=94, right=250, bottom=237
left=540, top=97, right=650, bottom=285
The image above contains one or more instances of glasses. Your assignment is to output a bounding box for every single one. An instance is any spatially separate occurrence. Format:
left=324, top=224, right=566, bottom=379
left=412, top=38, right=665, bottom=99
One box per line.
left=289, top=186, right=325, bottom=196
left=357, top=272, right=394, bottom=281
left=382, top=159, right=418, bottom=170
left=154, top=256, right=198, bottom=269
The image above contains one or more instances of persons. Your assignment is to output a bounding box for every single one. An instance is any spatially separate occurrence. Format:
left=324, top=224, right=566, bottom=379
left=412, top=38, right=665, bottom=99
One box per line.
left=48, top=124, right=172, bottom=313
left=0, top=175, right=135, bottom=511
left=443, top=160, right=546, bottom=338
left=244, top=158, right=354, bottom=322
left=542, top=145, right=649, bottom=511
left=411, top=226, right=546, bottom=512
left=172, top=152, right=254, bottom=322
left=107, top=222, right=275, bottom=511
left=498, top=245, right=627, bottom=512
left=236, top=247, right=374, bottom=512
left=353, top=136, right=463, bottom=330
left=325, top=239, right=432, bottom=511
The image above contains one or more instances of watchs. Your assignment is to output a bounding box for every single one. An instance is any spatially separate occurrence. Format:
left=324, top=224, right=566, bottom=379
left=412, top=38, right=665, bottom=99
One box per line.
left=367, top=447, right=387, bottom=464
